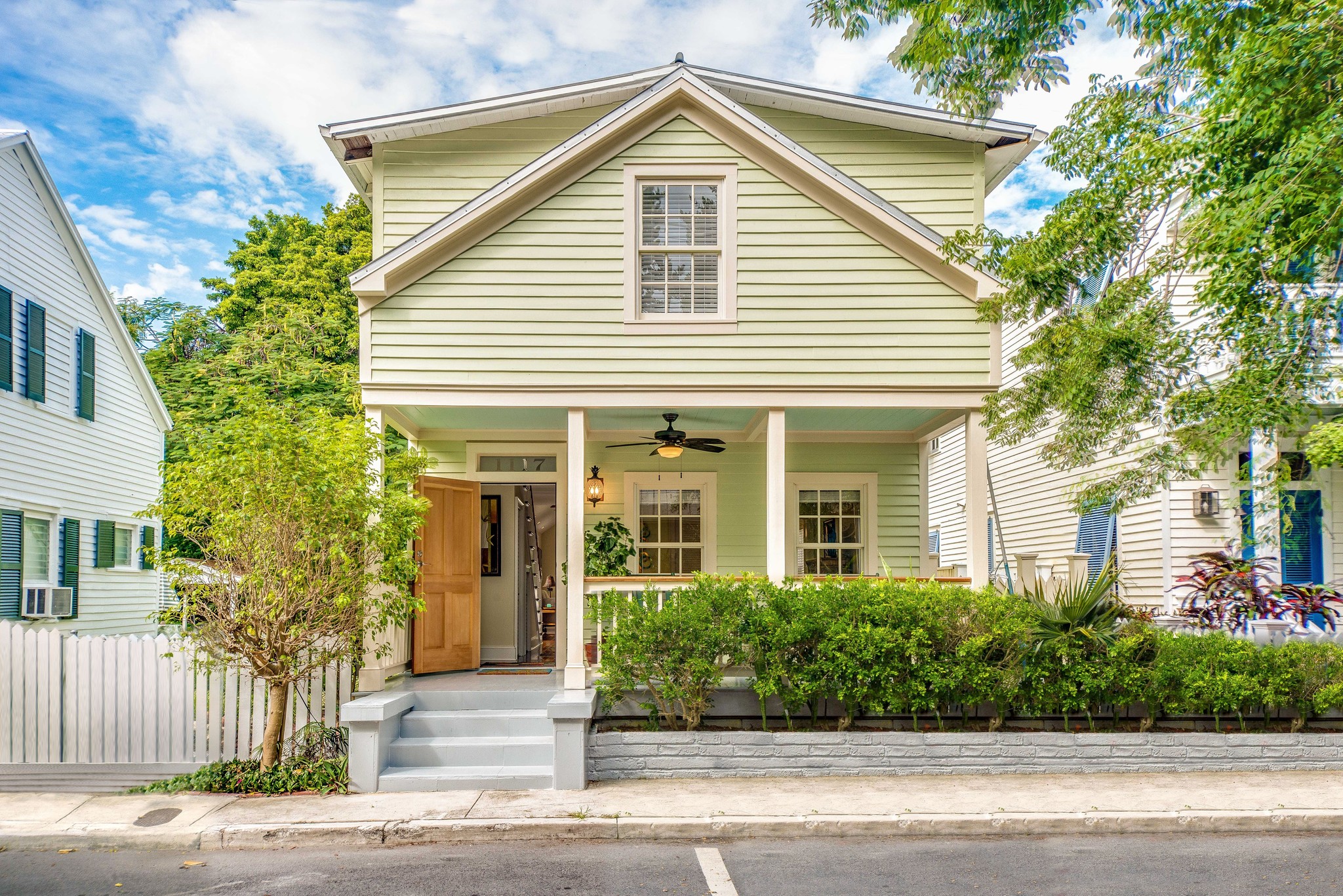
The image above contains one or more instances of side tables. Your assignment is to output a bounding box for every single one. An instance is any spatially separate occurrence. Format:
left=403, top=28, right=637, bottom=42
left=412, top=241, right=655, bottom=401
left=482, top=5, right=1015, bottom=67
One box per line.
left=541, top=608, right=556, bottom=636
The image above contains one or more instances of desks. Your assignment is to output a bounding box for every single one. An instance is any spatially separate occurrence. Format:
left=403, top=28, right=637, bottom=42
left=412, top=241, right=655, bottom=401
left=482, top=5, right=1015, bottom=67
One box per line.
left=805, top=557, right=851, bottom=573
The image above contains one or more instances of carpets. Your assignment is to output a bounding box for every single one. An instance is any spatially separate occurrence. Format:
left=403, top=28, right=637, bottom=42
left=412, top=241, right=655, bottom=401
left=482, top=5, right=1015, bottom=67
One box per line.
left=477, top=669, right=552, bottom=675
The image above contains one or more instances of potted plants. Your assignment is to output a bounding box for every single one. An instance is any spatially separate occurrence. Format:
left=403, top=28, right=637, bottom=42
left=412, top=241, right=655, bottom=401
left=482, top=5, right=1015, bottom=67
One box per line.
left=561, top=516, right=637, bottom=667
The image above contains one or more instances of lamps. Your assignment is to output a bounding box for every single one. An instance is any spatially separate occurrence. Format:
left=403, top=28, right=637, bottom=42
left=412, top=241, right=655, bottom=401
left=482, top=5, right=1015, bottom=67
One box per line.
left=1233, top=505, right=1248, bottom=518
left=542, top=575, right=555, bottom=598
left=658, top=445, right=683, bottom=482
left=586, top=465, right=604, bottom=508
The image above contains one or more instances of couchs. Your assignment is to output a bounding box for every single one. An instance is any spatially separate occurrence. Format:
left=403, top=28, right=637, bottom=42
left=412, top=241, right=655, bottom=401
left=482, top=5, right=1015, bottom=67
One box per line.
left=542, top=590, right=556, bottom=635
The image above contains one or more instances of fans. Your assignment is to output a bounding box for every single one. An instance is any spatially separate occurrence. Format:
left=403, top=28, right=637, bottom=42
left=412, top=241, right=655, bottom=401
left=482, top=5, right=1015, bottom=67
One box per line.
left=605, top=413, right=726, bottom=457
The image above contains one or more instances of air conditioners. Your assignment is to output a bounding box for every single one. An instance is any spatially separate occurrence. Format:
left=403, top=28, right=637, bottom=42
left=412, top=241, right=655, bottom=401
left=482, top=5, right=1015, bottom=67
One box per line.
left=22, top=587, right=73, bottom=618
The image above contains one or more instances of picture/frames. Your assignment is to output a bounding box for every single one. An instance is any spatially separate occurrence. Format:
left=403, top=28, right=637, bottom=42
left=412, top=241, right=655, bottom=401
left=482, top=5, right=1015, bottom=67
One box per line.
left=480, top=494, right=502, bottom=577
